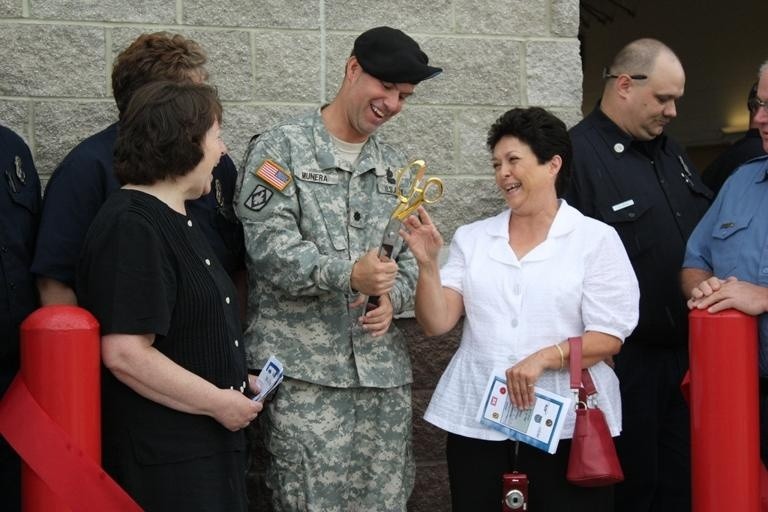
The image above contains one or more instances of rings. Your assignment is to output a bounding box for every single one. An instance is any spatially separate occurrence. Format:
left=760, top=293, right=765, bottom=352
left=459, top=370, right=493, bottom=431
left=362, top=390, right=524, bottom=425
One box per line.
left=525, top=382, right=534, bottom=387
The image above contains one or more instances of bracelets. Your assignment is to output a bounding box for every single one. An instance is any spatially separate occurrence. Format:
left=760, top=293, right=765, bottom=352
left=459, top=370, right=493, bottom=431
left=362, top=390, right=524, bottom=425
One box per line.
left=555, top=343, right=564, bottom=372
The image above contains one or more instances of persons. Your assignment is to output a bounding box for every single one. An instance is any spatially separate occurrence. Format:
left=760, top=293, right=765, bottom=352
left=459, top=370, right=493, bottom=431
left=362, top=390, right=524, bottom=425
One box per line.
left=227, top=24, right=421, bottom=511
left=677, top=59, right=767, bottom=394
left=73, top=77, right=264, bottom=509
left=396, top=105, right=642, bottom=508
left=29, top=27, right=242, bottom=313
left=560, top=34, right=715, bottom=510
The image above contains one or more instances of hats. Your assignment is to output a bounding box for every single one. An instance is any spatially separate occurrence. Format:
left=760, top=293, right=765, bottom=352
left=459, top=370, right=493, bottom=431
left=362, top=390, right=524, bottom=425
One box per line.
left=354, top=26, right=443, bottom=85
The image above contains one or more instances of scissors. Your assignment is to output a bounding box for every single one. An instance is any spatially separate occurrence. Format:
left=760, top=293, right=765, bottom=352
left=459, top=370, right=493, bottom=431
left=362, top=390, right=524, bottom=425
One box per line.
left=361, top=159, right=445, bottom=317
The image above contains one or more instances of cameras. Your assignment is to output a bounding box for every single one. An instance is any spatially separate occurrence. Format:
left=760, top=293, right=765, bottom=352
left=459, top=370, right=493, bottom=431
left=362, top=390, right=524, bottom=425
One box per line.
left=501, top=472, right=529, bottom=512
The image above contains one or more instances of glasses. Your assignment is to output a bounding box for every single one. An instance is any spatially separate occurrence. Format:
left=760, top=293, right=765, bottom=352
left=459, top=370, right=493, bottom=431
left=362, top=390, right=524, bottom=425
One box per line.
left=602, top=65, right=647, bottom=79
left=749, top=97, right=768, bottom=113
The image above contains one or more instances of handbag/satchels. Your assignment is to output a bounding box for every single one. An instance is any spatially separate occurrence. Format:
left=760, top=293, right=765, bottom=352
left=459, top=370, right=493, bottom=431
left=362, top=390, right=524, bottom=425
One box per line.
left=567, top=336, right=624, bottom=488
